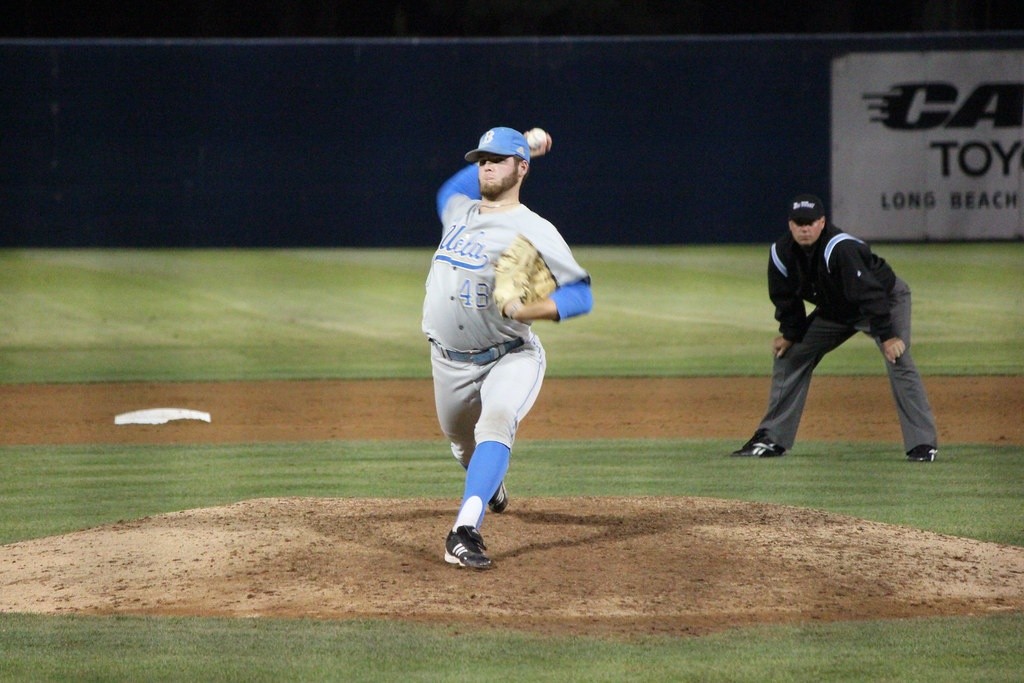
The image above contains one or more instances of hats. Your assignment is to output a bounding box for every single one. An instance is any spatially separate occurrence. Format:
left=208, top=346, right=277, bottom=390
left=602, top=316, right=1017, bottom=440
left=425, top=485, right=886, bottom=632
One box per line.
left=789, top=194, right=825, bottom=220
left=465, top=126, right=530, bottom=164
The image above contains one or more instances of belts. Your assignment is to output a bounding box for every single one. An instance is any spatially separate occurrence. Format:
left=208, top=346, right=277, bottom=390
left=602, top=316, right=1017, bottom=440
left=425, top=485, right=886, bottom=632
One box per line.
left=431, top=338, right=523, bottom=365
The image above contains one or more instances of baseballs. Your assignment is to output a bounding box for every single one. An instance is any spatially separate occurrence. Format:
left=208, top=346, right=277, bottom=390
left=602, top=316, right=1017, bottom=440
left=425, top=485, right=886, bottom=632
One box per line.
left=527, top=127, right=549, bottom=151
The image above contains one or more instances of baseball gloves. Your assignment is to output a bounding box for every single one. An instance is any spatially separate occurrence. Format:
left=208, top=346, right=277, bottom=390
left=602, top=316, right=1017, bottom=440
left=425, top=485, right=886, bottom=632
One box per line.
left=491, top=234, right=557, bottom=315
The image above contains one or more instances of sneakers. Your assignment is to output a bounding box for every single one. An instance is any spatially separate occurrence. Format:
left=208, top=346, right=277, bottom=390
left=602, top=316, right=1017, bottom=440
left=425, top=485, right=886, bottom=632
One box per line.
left=907, top=446, right=937, bottom=462
left=488, top=481, right=508, bottom=514
left=730, top=437, right=787, bottom=456
left=444, top=526, right=492, bottom=569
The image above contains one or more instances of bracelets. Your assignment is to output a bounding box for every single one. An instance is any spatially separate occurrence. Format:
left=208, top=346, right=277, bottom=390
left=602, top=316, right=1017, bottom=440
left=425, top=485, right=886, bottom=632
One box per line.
left=506, top=301, right=522, bottom=321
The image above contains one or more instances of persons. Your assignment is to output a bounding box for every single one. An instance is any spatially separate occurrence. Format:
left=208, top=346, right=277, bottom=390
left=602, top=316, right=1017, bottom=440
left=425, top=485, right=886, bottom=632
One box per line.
left=729, top=194, right=940, bottom=465
left=417, top=127, right=595, bottom=573
